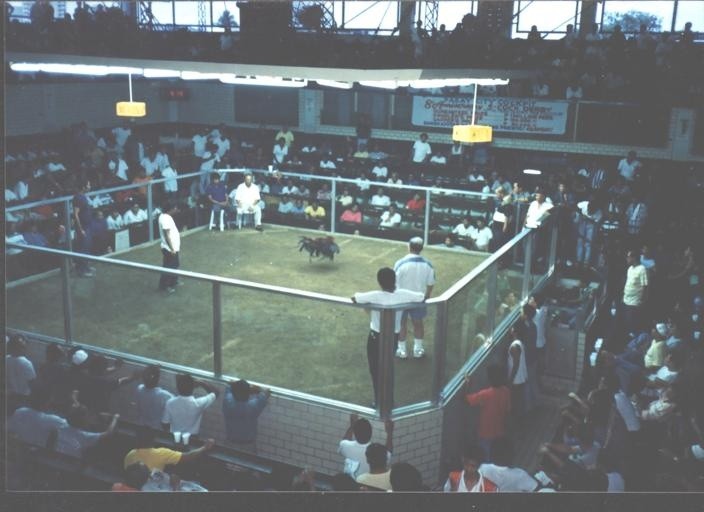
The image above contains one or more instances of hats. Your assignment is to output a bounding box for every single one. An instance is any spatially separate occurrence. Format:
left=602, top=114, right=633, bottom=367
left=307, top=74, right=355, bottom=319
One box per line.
left=656, top=323, right=668, bottom=337
left=71, top=350, right=88, bottom=367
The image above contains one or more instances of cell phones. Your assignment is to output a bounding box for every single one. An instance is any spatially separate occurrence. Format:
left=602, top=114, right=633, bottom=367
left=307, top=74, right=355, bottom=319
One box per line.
left=350, top=414, right=358, bottom=427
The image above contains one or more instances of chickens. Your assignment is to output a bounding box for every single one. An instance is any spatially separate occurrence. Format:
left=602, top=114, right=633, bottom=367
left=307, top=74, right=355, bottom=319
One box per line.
left=296, top=235, right=339, bottom=264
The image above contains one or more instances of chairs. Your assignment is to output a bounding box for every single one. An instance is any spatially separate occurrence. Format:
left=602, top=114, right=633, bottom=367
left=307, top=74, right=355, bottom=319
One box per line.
left=548, top=274, right=702, bottom=492
left=411, top=141, right=664, bottom=272
left=235, top=123, right=409, bottom=244
left=0, top=122, right=234, bottom=271
left=2, top=391, right=375, bottom=492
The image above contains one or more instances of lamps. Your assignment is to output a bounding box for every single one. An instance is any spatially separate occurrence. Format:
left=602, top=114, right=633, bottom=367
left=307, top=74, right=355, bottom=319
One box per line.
left=116, top=73, right=145, bottom=119
left=450, top=83, right=493, bottom=142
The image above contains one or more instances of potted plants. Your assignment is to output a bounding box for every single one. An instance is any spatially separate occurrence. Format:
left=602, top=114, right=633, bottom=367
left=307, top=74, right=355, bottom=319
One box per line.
left=297, top=236, right=339, bottom=268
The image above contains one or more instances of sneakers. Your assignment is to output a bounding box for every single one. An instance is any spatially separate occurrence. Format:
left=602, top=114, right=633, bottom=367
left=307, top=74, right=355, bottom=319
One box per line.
left=414, top=347, right=425, bottom=358
left=82, top=267, right=96, bottom=277
left=165, top=286, right=175, bottom=294
left=395, top=349, right=408, bottom=358
left=176, top=281, right=184, bottom=286
